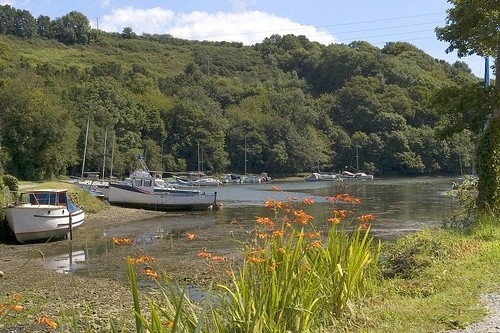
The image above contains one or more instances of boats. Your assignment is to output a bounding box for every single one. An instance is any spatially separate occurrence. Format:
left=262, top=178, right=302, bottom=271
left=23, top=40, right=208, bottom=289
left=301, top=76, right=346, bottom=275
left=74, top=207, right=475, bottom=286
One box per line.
left=303, top=142, right=374, bottom=182
left=4, top=189, right=85, bottom=243
left=172, top=136, right=271, bottom=186
left=24, top=247, right=87, bottom=276
left=106, top=169, right=217, bottom=212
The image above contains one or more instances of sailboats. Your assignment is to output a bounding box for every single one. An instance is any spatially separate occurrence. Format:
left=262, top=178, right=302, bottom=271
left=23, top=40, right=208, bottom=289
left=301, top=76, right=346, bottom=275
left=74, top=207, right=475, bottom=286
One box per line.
left=62, top=118, right=111, bottom=195
